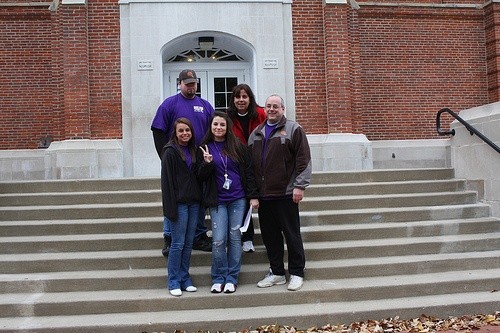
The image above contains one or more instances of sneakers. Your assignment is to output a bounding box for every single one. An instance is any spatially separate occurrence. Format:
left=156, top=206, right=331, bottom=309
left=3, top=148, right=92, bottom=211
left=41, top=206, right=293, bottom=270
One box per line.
left=224, top=283, right=236, bottom=293
left=288, top=274, right=303, bottom=290
left=257, top=268, right=286, bottom=288
left=210, top=283, right=224, bottom=293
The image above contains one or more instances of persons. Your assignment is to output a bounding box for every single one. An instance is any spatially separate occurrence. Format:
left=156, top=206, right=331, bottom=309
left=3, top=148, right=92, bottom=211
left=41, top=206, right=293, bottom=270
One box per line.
left=246, top=94, right=312, bottom=291
left=223, top=83, right=268, bottom=253
left=160, top=117, right=202, bottom=297
left=151, top=68, right=215, bottom=257
left=195, top=110, right=259, bottom=293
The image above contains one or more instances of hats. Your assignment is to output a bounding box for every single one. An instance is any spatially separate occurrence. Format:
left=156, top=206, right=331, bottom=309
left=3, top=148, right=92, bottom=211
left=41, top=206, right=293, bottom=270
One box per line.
left=179, top=68, right=199, bottom=85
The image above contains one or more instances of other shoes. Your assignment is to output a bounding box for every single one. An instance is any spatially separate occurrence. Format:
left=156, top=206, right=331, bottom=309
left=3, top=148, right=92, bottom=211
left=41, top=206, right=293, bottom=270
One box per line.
left=242, top=241, right=255, bottom=252
left=185, top=286, right=198, bottom=292
left=162, top=233, right=172, bottom=255
left=192, top=232, right=212, bottom=252
left=169, top=289, right=182, bottom=296
